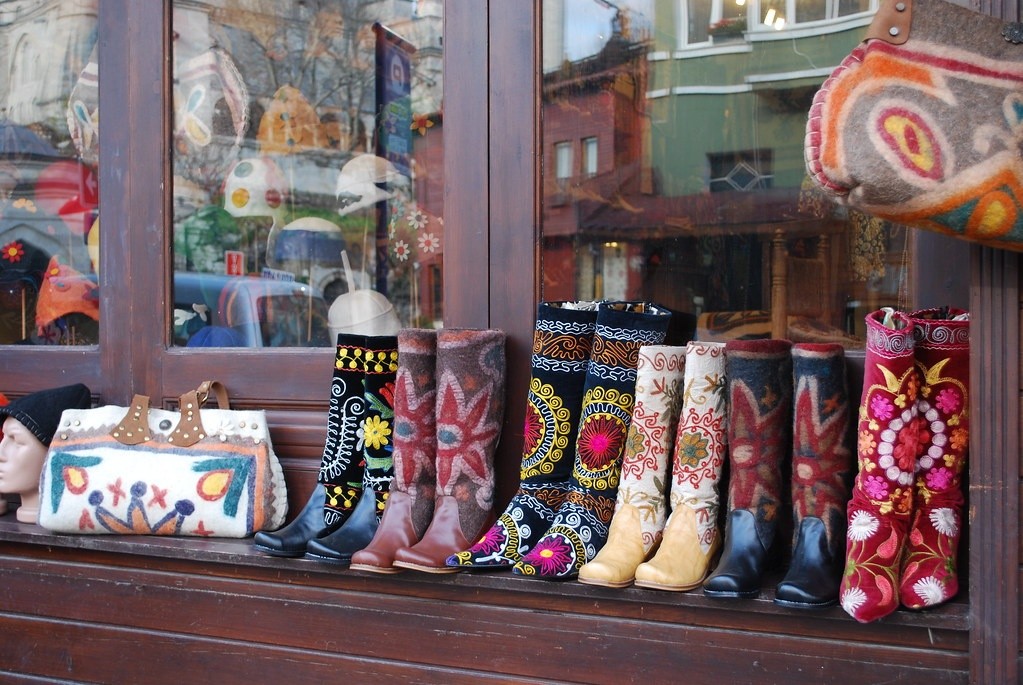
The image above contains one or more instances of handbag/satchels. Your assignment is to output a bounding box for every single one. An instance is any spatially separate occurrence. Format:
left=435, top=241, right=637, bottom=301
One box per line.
left=36, top=379, right=289, bottom=537
left=803, top=1, right=1023, bottom=253
left=360, top=189, right=442, bottom=278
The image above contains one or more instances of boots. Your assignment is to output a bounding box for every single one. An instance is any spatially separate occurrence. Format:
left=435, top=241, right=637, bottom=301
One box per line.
left=348, top=327, right=437, bottom=576
left=391, top=327, right=508, bottom=574
left=578, top=345, right=686, bottom=588
left=703, top=338, right=793, bottom=597
left=773, top=341, right=852, bottom=610
left=634, top=338, right=727, bottom=592
left=899, top=303, right=969, bottom=611
left=512, top=297, right=672, bottom=581
left=840, top=307, right=919, bottom=623
left=444, top=295, right=596, bottom=567
left=247, top=332, right=365, bottom=556
left=305, top=332, right=398, bottom=564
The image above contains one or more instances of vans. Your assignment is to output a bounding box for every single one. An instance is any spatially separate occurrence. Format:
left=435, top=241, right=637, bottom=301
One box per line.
left=172, top=268, right=332, bottom=349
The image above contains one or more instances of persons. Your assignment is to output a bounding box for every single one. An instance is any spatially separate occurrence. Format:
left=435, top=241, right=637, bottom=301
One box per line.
left=275, top=0, right=371, bottom=109
left=0, top=383, right=92, bottom=523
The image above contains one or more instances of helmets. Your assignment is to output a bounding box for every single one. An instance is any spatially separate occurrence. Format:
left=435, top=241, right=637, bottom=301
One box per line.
left=336, top=153, right=413, bottom=216
left=224, top=159, right=289, bottom=217
left=273, top=216, right=344, bottom=278
left=328, top=290, right=405, bottom=347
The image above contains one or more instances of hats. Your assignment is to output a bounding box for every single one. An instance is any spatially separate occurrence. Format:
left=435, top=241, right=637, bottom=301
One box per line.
left=0, top=382, right=93, bottom=449
left=36, top=253, right=100, bottom=334
left=35, top=161, right=98, bottom=216
